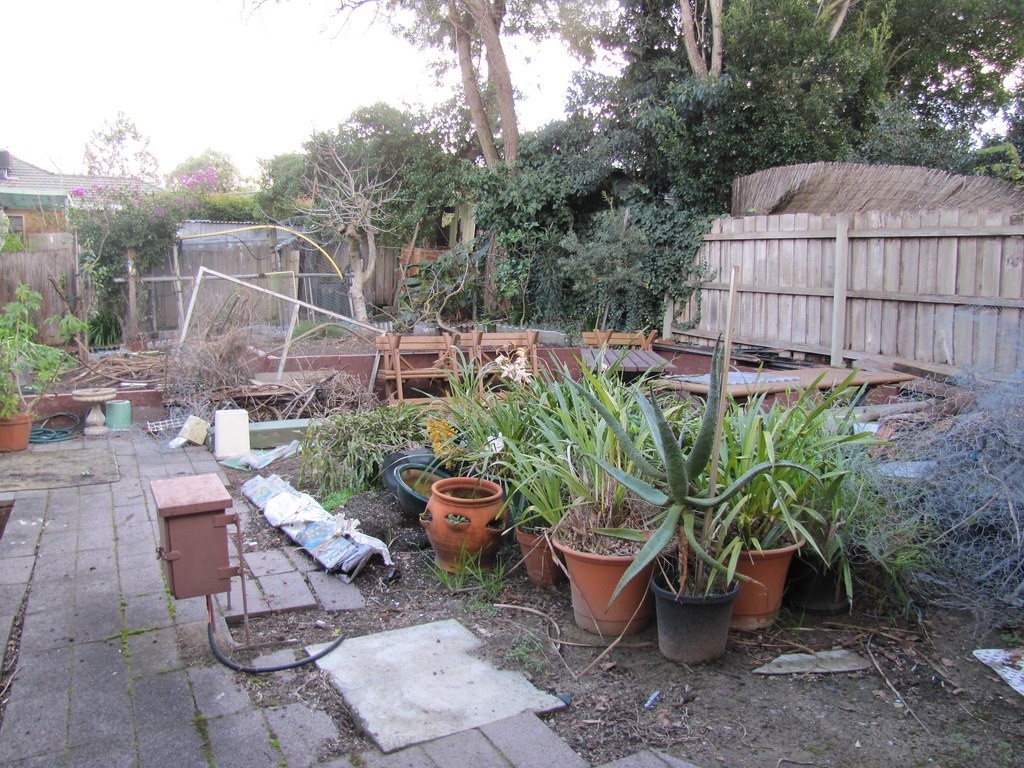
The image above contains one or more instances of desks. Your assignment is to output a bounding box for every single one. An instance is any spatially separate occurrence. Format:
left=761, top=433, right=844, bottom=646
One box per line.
left=650, top=365, right=914, bottom=406
left=579, top=348, right=676, bottom=385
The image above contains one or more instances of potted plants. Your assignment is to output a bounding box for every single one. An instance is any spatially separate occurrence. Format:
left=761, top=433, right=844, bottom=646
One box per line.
left=386, top=349, right=897, bottom=660
left=0, top=281, right=86, bottom=454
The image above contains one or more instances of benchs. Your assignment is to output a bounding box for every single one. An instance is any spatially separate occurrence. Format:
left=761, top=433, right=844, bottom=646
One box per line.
left=580, top=329, right=658, bottom=352
left=377, top=328, right=541, bottom=408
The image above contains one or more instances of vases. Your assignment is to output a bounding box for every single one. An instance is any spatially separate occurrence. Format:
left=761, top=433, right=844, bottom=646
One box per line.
left=394, top=462, right=454, bottom=518
left=418, top=478, right=508, bottom=575
